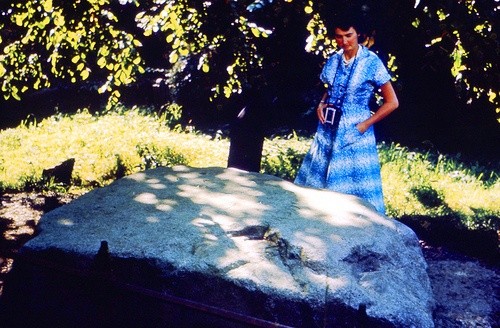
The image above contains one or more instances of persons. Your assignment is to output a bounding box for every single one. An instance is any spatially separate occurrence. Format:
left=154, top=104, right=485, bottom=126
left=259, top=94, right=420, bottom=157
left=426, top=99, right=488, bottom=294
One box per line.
left=293, top=16, right=400, bottom=217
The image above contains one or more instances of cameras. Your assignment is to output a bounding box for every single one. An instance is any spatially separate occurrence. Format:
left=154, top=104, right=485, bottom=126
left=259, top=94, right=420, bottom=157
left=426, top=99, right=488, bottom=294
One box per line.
left=323, top=106, right=342, bottom=128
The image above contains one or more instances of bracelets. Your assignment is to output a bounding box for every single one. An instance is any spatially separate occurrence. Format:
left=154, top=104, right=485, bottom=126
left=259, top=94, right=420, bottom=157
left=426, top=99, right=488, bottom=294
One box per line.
left=321, top=100, right=324, bottom=104
